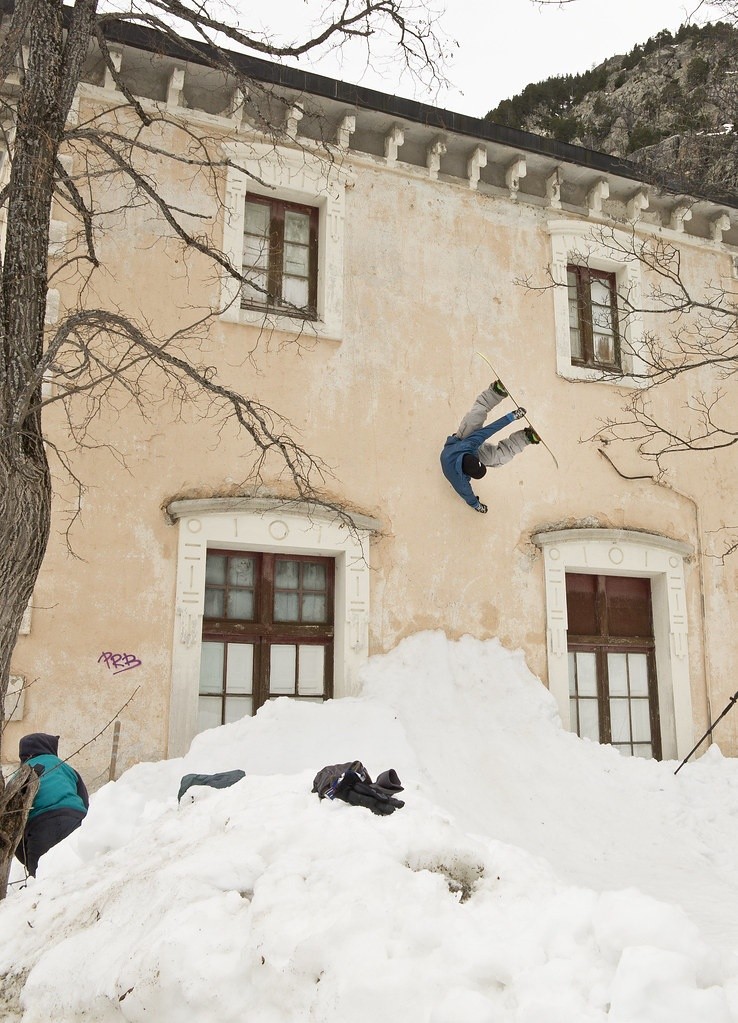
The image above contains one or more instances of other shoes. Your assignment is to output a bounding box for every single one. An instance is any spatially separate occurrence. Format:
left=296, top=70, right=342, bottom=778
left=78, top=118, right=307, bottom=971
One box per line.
left=492, top=380, right=507, bottom=398
left=524, top=426, right=540, bottom=446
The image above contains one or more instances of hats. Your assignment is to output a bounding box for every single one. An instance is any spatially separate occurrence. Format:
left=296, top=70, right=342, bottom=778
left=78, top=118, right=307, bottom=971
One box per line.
left=461, top=453, right=487, bottom=479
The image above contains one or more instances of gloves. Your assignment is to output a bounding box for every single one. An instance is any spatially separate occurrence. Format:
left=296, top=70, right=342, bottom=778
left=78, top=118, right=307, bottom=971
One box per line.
left=473, top=495, right=488, bottom=515
left=512, top=406, right=527, bottom=420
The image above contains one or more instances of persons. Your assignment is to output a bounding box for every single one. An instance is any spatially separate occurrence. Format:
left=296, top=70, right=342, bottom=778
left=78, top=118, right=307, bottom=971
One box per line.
left=439, top=379, right=541, bottom=514
left=8, top=732, right=88, bottom=892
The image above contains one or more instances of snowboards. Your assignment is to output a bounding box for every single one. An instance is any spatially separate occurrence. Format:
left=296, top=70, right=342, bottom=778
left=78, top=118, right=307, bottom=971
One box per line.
left=475, top=351, right=559, bottom=470
